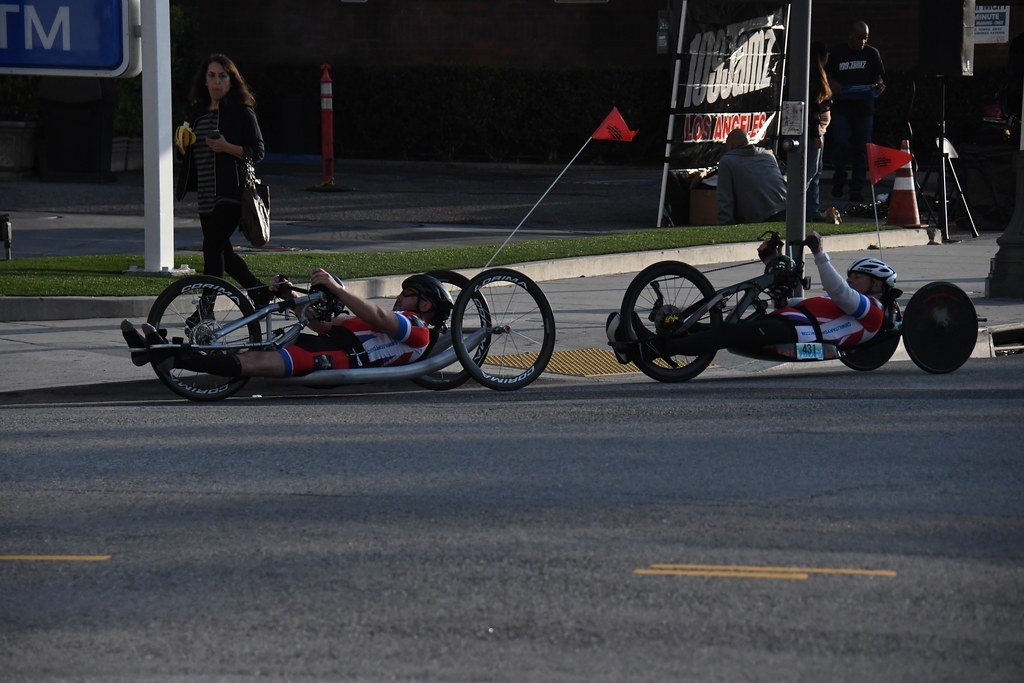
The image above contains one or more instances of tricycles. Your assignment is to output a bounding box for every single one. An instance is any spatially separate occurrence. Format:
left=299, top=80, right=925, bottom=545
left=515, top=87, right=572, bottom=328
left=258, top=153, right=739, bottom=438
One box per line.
left=129, top=266, right=558, bottom=401
left=604, top=229, right=989, bottom=382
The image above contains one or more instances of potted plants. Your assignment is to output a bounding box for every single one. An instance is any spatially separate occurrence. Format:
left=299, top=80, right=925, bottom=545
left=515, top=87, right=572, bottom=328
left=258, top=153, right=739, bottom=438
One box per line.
left=0, top=73, right=42, bottom=180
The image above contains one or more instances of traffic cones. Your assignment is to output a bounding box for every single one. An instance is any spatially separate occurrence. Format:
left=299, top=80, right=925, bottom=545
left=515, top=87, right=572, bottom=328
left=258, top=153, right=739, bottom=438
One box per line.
left=883, top=140, right=931, bottom=230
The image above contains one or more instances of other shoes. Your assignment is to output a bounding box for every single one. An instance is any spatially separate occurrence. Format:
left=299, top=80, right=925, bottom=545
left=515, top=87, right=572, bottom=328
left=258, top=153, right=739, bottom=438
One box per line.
left=849, top=192, right=865, bottom=202
left=831, top=173, right=844, bottom=197
left=807, top=216, right=826, bottom=222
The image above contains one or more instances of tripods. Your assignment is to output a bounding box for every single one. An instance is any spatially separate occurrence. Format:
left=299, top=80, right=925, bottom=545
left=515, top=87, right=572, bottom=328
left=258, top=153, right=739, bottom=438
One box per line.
left=917, top=75, right=979, bottom=240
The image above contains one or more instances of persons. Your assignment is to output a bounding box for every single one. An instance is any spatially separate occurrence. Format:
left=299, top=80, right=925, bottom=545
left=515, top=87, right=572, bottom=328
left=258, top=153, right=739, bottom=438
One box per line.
left=605, top=230, right=897, bottom=365
left=175, top=53, right=275, bottom=326
left=120, top=268, right=456, bottom=378
left=806, top=40, right=842, bottom=223
left=715, top=128, right=788, bottom=226
left=827, top=20, right=886, bottom=203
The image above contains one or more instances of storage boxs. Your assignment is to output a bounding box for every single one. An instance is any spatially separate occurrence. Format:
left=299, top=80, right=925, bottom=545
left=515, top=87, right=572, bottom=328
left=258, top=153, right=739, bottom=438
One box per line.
left=689, top=189, right=718, bottom=224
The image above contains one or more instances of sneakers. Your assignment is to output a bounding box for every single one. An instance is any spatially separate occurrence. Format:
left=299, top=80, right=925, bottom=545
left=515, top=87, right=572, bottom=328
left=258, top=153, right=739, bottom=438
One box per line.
left=630, top=311, right=661, bottom=363
left=606, top=311, right=632, bottom=364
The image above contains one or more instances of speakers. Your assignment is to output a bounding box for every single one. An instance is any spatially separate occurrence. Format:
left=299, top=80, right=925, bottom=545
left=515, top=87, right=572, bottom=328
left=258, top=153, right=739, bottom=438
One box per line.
left=918, top=0, right=965, bottom=76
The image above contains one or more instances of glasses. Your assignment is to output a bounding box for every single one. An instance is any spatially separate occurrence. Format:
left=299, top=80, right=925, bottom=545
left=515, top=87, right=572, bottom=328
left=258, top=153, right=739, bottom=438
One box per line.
left=403, top=287, right=426, bottom=303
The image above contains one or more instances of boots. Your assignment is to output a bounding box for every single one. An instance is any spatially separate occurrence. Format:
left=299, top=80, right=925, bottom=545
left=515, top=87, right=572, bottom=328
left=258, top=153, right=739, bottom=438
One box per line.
left=248, top=279, right=275, bottom=319
left=186, top=298, right=216, bottom=326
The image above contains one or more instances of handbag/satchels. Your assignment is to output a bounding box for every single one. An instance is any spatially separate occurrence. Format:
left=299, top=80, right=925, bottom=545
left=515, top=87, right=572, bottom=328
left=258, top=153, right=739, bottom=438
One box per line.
left=238, top=149, right=271, bottom=247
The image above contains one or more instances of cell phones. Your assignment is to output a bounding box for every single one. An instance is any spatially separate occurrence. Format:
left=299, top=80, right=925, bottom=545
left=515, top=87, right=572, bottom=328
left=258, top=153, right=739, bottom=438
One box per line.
left=208, top=130, right=220, bottom=139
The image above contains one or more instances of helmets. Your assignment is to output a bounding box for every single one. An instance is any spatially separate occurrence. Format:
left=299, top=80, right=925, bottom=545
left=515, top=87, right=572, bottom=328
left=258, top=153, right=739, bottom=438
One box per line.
left=402, top=274, right=455, bottom=311
left=846, top=257, right=898, bottom=289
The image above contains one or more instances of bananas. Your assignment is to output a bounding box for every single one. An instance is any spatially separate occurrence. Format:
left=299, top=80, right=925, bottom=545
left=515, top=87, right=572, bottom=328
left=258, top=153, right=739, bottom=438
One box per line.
left=175, top=121, right=196, bottom=154
